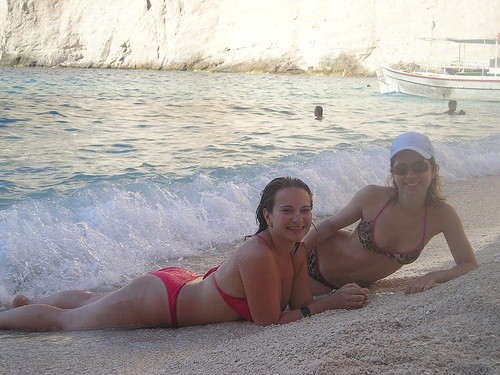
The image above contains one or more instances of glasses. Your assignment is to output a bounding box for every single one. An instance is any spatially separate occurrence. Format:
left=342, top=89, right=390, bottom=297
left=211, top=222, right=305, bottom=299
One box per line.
left=391, top=161, right=429, bottom=175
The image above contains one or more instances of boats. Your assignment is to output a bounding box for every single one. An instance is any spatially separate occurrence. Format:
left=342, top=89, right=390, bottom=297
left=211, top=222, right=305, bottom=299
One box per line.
left=376, top=36, right=500, bottom=101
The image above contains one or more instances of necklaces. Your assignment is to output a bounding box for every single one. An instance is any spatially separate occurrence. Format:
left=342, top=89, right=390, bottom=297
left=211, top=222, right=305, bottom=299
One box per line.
left=444, top=100, right=465, bottom=114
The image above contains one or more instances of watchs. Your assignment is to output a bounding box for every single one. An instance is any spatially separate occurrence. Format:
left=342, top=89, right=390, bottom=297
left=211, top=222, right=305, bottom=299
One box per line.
left=300, top=305, right=310, bottom=317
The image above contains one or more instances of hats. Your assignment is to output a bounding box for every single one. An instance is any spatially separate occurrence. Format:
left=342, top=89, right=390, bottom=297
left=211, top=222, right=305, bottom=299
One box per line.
left=389, top=132, right=434, bottom=161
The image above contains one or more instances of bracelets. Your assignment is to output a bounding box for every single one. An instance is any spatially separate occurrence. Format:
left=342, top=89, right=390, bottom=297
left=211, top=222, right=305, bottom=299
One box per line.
left=329, top=289, right=337, bottom=294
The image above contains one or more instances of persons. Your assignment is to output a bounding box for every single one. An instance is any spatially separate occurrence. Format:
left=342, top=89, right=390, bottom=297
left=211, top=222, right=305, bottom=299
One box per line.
left=313, top=106, right=324, bottom=120
left=0, top=178, right=368, bottom=329
left=300, top=131, right=479, bottom=296
